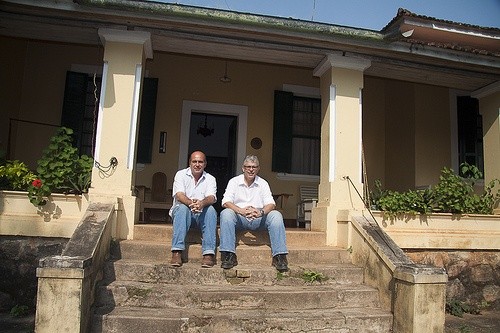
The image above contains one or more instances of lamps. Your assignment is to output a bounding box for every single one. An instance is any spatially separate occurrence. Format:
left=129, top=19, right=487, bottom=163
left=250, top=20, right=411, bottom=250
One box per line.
left=197, top=115, right=215, bottom=138
left=219, top=60, right=230, bottom=83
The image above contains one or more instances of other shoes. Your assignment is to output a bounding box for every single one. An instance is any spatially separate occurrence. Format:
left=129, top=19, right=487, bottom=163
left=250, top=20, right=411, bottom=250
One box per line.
left=201, top=254, right=215, bottom=268
left=170, top=250, right=182, bottom=267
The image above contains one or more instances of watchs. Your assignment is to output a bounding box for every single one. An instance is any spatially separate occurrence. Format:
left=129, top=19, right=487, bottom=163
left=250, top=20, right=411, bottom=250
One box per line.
left=260, top=209, right=264, bottom=215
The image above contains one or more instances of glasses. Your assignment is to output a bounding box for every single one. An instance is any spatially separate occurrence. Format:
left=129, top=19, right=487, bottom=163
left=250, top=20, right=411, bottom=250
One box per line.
left=244, top=165, right=259, bottom=169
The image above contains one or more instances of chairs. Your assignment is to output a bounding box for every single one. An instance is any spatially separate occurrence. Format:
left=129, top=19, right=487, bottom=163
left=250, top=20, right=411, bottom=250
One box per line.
left=272, top=191, right=293, bottom=213
left=136, top=172, right=172, bottom=223
left=297, top=185, right=319, bottom=229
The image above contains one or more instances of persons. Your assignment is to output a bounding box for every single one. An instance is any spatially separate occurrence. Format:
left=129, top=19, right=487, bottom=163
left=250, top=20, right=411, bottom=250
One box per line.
left=167, top=150, right=217, bottom=268
left=220, top=155, right=288, bottom=270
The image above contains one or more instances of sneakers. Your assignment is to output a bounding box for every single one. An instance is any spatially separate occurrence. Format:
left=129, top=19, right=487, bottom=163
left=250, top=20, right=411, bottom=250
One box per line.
left=220, top=251, right=238, bottom=269
left=272, top=254, right=289, bottom=270
